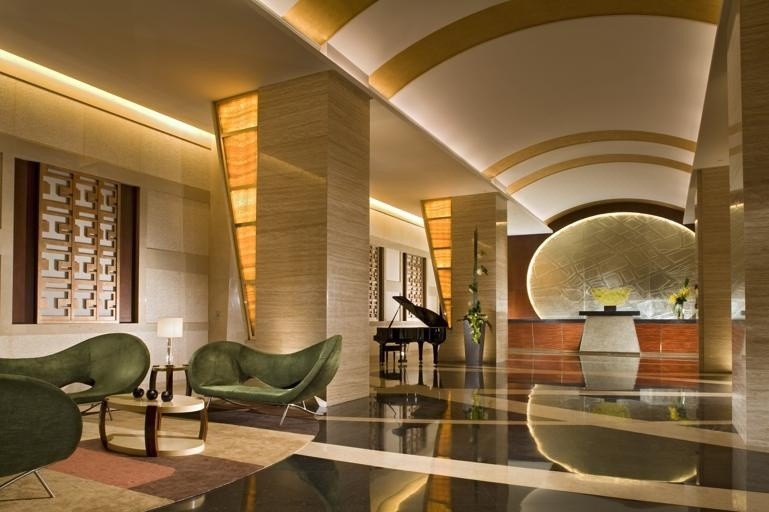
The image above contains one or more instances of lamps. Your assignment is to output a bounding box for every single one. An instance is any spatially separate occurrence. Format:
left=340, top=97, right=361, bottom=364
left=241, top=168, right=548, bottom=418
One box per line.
left=157, top=315, right=184, bottom=366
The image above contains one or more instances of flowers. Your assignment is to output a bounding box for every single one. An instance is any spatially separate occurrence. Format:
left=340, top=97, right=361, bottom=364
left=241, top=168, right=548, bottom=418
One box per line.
left=670, top=287, right=691, bottom=303
left=668, top=390, right=690, bottom=426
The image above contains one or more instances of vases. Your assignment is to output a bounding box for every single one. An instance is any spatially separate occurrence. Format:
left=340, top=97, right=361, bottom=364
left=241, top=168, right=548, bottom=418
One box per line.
left=679, top=387, right=687, bottom=406
left=674, top=301, right=684, bottom=319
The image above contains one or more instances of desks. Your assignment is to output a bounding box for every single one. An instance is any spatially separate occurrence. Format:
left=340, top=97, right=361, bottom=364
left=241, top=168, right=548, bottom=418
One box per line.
left=149, top=364, right=191, bottom=397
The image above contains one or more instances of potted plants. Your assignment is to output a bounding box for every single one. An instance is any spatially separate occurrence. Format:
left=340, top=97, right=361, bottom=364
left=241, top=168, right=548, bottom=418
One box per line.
left=461, top=301, right=492, bottom=365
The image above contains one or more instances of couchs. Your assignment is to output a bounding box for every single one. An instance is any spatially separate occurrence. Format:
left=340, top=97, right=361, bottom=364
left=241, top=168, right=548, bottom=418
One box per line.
left=186, top=334, right=352, bottom=425
left=0, top=332, right=150, bottom=500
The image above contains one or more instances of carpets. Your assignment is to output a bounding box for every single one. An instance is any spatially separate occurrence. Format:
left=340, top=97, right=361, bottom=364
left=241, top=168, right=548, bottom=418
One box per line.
left=2, top=377, right=320, bottom=512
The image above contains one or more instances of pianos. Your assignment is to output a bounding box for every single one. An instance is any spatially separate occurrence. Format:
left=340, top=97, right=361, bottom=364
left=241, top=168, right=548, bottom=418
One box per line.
left=373, top=296, right=448, bottom=367
